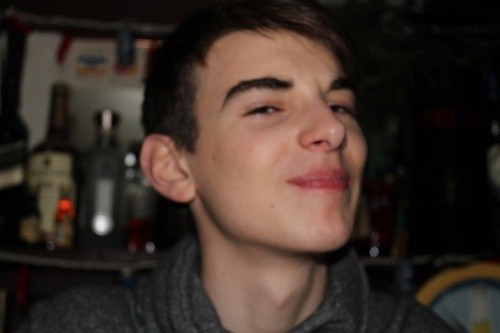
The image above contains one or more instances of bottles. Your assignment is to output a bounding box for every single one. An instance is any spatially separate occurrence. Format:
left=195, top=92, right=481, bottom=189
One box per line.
left=118, top=145, right=162, bottom=256
left=18, top=81, right=85, bottom=256
left=76, top=108, right=126, bottom=255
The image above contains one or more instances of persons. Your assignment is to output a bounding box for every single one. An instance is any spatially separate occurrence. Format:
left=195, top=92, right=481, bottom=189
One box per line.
left=25, top=6, right=464, bottom=332
